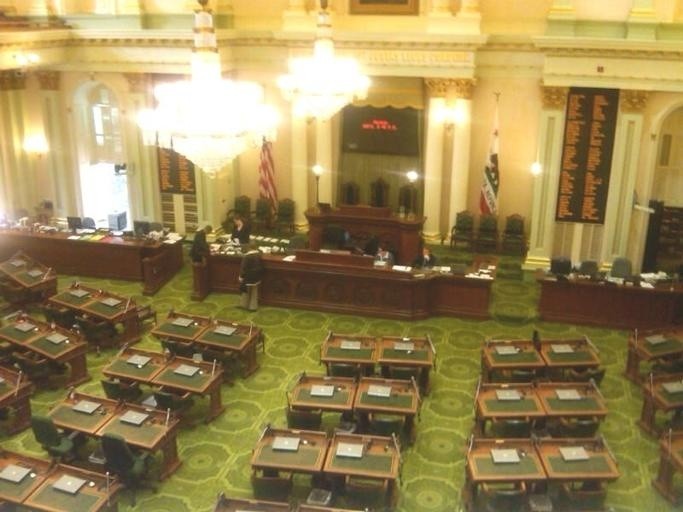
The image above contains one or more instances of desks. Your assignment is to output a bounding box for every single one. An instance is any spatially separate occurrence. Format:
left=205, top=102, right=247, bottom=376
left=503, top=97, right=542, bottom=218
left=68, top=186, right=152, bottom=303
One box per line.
left=2, top=214, right=682, bottom=329
left=624, top=329, right=682, bottom=504
left=464, top=336, right=621, bottom=508
left=214, top=330, right=439, bottom=507
left=0, top=308, right=263, bottom=512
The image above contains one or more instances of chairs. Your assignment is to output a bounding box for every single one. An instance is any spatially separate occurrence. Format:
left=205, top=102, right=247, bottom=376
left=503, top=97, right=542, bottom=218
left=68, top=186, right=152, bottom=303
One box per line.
left=228, top=196, right=298, bottom=229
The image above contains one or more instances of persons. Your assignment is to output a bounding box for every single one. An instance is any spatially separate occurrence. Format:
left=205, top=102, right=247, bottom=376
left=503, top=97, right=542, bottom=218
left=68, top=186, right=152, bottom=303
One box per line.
left=376, top=244, right=394, bottom=265
left=231, top=215, right=249, bottom=245
left=419, top=247, right=434, bottom=266
left=189, top=226, right=212, bottom=264
left=238, top=242, right=263, bottom=298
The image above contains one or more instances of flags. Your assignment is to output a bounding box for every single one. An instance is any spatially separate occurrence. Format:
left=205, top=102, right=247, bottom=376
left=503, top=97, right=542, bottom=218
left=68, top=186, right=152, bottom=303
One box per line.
left=257, top=139, right=279, bottom=222
left=480, top=110, right=499, bottom=217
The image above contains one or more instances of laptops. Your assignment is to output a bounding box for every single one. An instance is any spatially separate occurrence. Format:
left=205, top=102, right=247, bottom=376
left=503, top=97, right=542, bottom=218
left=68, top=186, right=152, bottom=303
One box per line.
left=310, top=384, right=334, bottom=396
left=336, top=442, right=364, bottom=458
left=73, top=400, right=102, bottom=414
left=1, top=257, right=122, bottom=382
left=213, top=324, right=237, bottom=335
left=173, top=363, right=200, bottom=377
left=171, top=317, right=194, bottom=327
left=367, top=384, right=392, bottom=397
left=393, top=342, right=415, bottom=351
left=119, top=409, right=149, bottom=425
left=488, top=333, right=683, bottom=463
left=1, top=463, right=31, bottom=483
left=272, top=436, right=300, bottom=451
left=340, top=340, right=361, bottom=350
left=53, top=474, right=87, bottom=494
left=126, top=353, right=151, bottom=366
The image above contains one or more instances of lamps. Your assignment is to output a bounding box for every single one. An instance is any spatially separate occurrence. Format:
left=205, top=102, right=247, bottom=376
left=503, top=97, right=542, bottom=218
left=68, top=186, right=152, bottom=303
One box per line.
left=406, top=170, right=418, bottom=222
left=138, top=0, right=284, bottom=144
left=267, top=0, right=370, bottom=120
left=312, top=165, right=323, bottom=216
